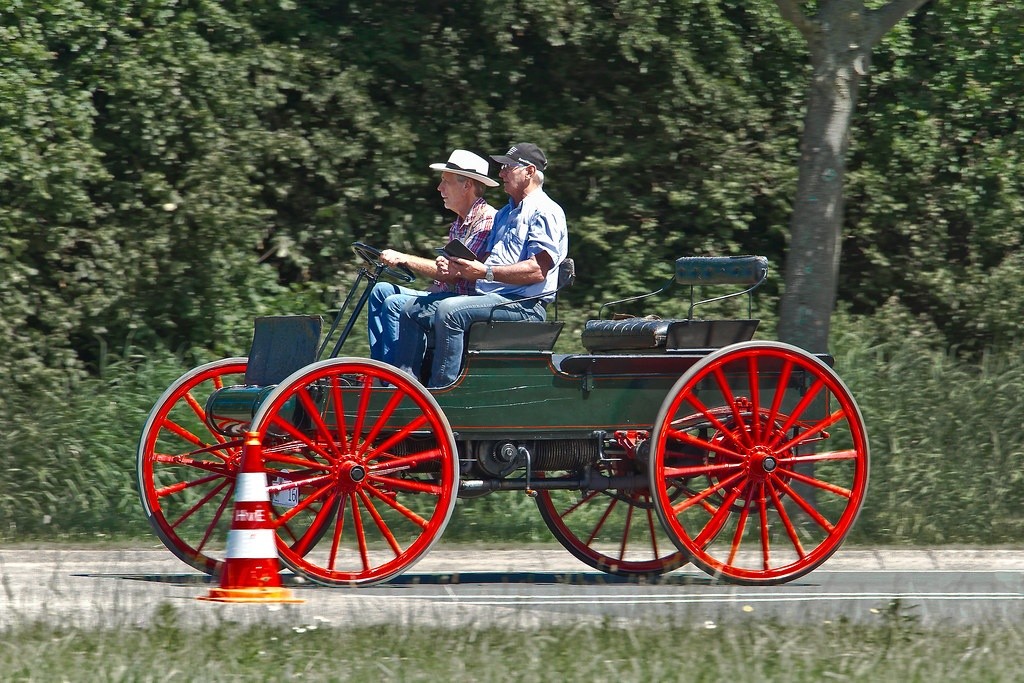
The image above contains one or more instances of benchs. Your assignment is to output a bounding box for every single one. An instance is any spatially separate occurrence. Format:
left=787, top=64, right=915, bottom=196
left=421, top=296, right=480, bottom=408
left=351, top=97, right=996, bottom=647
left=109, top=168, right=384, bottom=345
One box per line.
left=581, top=254, right=769, bottom=355
left=427, top=258, right=576, bottom=351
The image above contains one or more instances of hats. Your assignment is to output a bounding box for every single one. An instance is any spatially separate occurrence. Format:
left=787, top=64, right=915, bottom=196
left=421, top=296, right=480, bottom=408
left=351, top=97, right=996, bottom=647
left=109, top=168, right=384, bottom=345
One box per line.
left=490, top=142, right=547, bottom=170
left=429, top=149, right=500, bottom=187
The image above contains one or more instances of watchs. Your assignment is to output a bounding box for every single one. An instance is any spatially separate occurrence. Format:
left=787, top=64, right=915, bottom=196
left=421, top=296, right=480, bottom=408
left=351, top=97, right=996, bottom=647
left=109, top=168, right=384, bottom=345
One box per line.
left=485, top=266, right=494, bottom=282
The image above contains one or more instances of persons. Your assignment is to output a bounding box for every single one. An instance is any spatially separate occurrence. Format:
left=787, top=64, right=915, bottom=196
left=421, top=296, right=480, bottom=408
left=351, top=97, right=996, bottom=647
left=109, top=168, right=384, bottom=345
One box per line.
left=368, top=148, right=500, bottom=386
left=387, top=142, right=568, bottom=387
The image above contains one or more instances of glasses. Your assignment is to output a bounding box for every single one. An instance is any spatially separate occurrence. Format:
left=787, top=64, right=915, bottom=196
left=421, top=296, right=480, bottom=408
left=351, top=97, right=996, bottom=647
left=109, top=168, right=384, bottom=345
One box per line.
left=499, top=163, right=527, bottom=170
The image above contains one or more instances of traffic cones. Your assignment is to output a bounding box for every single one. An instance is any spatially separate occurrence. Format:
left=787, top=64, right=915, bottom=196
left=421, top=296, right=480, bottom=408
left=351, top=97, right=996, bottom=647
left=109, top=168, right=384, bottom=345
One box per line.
left=195, top=430, right=307, bottom=604
